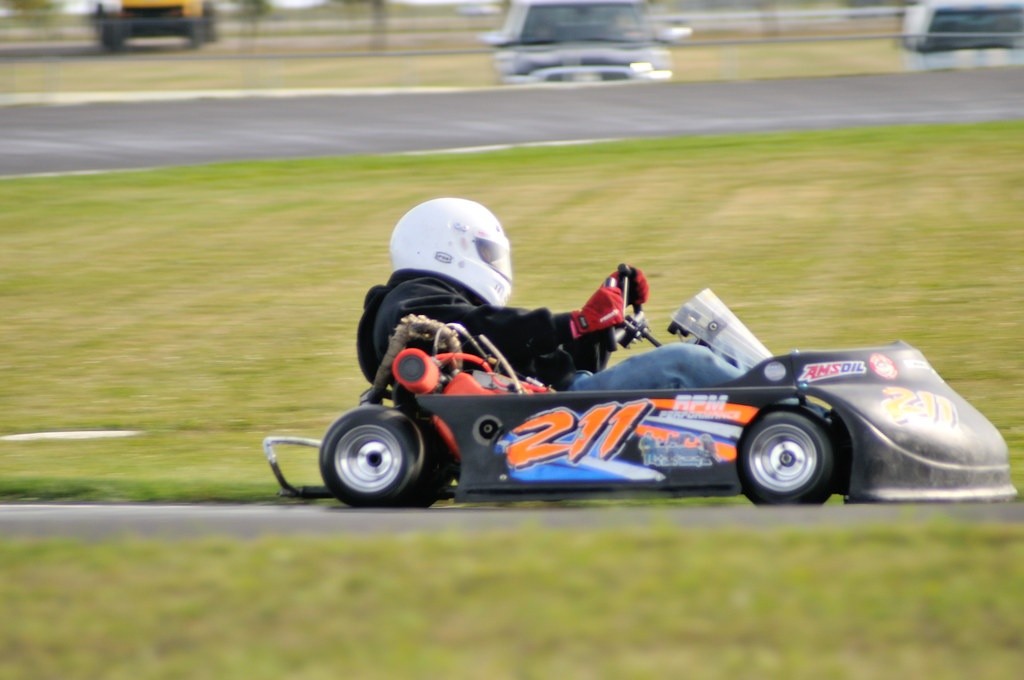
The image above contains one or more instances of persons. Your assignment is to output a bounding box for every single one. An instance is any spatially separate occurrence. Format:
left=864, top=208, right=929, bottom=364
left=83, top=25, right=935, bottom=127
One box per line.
left=357, top=199, right=748, bottom=391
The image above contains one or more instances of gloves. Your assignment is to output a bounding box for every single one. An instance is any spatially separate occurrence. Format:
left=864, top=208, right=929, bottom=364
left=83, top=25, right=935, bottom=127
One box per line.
left=568, top=263, right=651, bottom=339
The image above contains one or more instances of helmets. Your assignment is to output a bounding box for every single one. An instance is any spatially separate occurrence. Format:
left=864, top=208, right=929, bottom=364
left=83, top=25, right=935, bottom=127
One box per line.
left=387, top=196, right=514, bottom=311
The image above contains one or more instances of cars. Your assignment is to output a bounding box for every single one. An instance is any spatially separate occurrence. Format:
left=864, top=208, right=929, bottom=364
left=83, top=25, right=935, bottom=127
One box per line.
left=898, top=1, right=1024, bottom=75
left=479, top=1, right=697, bottom=88
